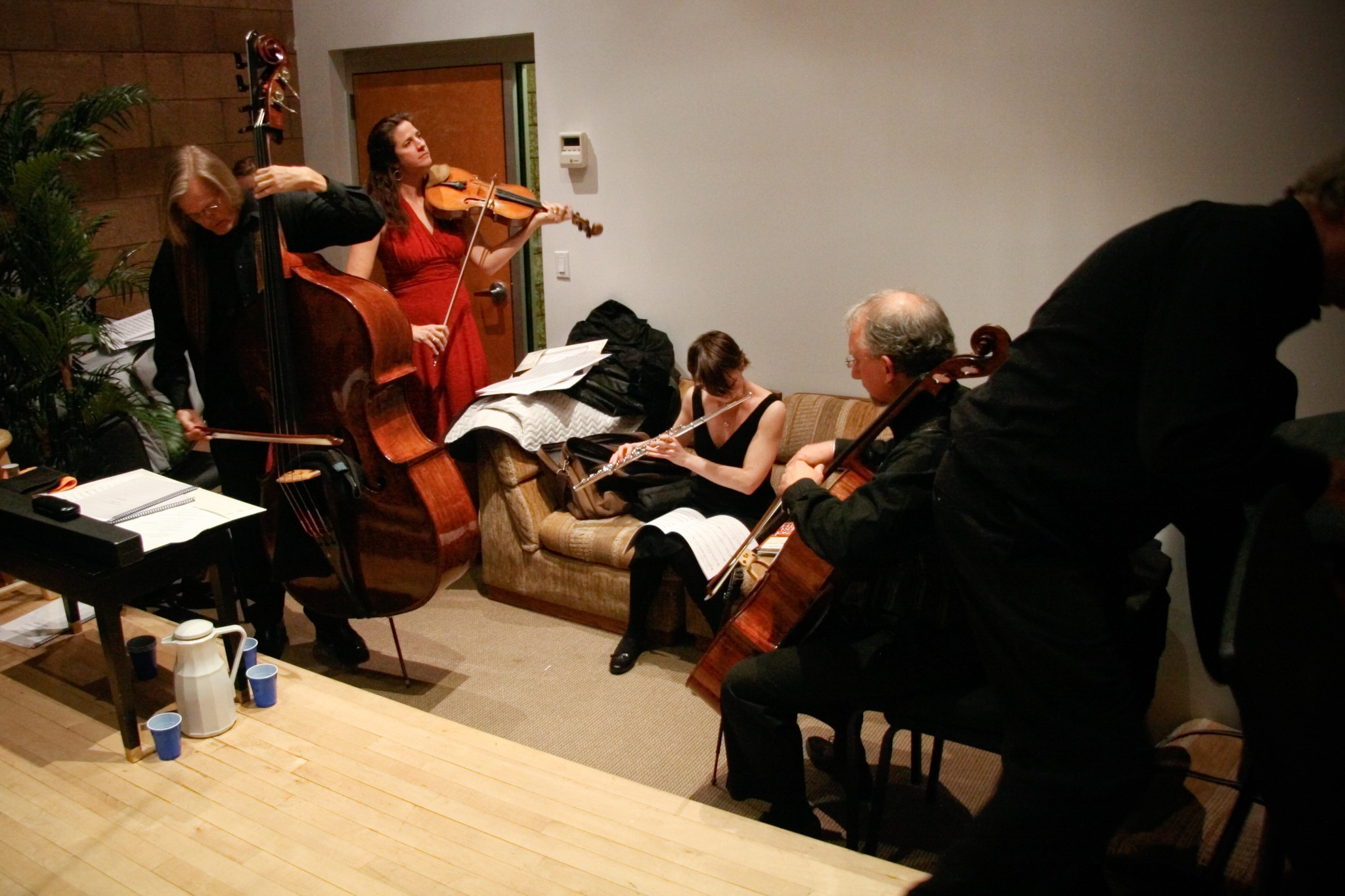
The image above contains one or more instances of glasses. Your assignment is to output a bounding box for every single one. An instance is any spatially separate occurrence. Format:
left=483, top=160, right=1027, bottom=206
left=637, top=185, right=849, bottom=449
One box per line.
left=180, top=193, right=221, bottom=221
left=845, top=354, right=882, bottom=369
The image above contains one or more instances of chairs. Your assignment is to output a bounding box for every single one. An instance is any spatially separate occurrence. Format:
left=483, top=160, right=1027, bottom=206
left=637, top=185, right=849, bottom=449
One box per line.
left=38, top=375, right=221, bottom=491
left=841, top=711, right=1004, bottom=855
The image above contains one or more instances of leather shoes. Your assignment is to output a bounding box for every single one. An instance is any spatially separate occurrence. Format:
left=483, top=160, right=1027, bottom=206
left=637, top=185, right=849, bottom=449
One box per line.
left=610, top=628, right=655, bottom=673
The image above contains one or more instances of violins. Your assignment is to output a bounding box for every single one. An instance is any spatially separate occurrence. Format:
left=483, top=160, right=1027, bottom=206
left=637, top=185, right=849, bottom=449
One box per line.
left=423, top=163, right=604, bottom=238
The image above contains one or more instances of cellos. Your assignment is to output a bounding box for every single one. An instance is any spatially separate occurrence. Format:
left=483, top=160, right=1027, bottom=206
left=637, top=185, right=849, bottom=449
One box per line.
left=235, top=26, right=483, bottom=681
left=684, top=322, right=1014, bottom=790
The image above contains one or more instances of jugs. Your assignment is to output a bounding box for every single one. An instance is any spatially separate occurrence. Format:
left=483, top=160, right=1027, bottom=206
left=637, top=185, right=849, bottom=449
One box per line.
left=161, top=618, right=247, bottom=738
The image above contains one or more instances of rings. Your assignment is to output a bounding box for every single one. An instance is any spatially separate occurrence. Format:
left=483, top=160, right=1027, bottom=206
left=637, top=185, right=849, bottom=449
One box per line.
left=553, top=210, right=557, bottom=213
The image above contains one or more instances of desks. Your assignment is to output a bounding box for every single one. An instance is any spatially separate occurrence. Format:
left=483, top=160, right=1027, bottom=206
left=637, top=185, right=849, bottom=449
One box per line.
left=0, top=466, right=263, bottom=763
left=0, top=579, right=932, bottom=896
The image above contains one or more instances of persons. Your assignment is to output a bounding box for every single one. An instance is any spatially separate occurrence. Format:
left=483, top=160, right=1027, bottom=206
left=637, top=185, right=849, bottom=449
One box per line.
left=344, top=113, right=571, bottom=569
left=934, top=145, right=1345, bottom=896
left=718, top=288, right=970, bottom=838
left=609, top=330, right=787, bottom=675
left=146, top=144, right=388, bottom=657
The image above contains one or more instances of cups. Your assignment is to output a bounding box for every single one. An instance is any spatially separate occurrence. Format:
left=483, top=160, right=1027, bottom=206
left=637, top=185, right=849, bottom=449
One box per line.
left=127, top=634, right=158, bottom=683
left=245, top=663, right=278, bottom=707
left=146, top=712, right=182, bottom=760
left=1, top=463, right=19, bottom=478
left=243, top=637, right=258, bottom=669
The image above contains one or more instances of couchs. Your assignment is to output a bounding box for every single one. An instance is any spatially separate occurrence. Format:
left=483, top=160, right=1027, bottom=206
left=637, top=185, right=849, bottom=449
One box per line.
left=478, top=378, right=893, bottom=650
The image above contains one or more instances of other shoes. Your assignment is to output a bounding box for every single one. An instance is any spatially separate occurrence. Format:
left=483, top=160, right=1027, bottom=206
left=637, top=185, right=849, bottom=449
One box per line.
left=806, top=736, right=872, bottom=791
left=315, top=621, right=370, bottom=665
left=756, top=809, right=820, bottom=836
left=253, top=621, right=290, bottom=661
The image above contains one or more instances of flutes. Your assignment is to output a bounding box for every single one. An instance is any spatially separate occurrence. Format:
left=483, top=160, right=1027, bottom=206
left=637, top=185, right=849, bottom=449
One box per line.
left=573, top=390, right=754, bottom=493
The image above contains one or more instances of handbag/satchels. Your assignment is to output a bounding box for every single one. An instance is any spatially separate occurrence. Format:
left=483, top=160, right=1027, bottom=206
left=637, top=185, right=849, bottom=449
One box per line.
left=536, top=431, right=708, bottom=522
